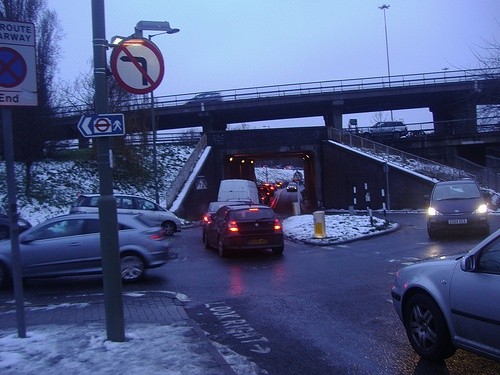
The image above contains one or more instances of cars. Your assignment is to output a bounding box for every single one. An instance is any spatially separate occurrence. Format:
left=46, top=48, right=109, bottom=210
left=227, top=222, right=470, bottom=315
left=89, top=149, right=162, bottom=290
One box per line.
left=287, top=181, right=298, bottom=192
left=389, top=229, right=500, bottom=361
left=0, top=193, right=183, bottom=289
left=185, top=92, right=223, bottom=106
left=259, top=181, right=283, bottom=201
left=426, top=178, right=489, bottom=236
left=202, top=199, right=285, bottom=257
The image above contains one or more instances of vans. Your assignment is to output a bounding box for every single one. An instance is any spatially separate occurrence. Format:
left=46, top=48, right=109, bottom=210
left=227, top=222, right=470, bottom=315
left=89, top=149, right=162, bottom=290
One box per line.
left=218, top=178, right=259, bottom=205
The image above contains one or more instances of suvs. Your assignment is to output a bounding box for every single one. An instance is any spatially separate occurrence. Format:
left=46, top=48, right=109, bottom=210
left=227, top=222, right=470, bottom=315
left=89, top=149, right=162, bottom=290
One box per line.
left=358, top=119, right=409, bottom=140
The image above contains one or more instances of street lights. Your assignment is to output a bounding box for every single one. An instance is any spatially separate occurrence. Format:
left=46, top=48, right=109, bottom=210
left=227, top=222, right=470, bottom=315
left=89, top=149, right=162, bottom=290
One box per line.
left=378, top=3, right=394, bottom=122
left=149, top=27, right=181, bottom=205
